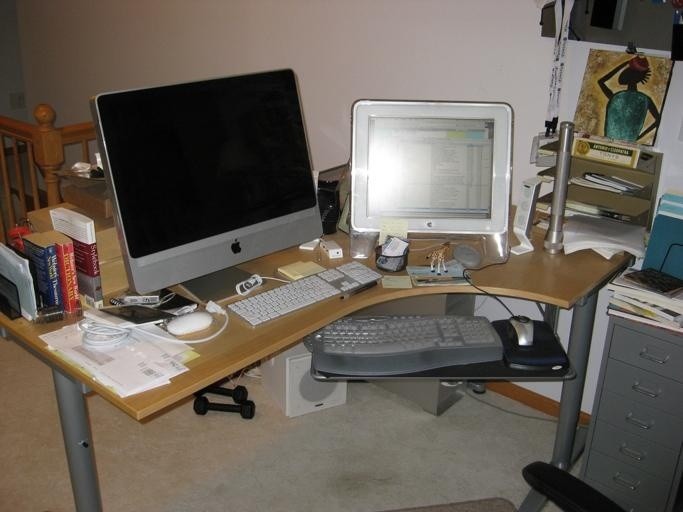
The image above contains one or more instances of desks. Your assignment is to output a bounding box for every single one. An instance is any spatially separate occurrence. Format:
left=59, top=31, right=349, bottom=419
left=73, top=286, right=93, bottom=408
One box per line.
left=0, top=203, right=637, bottom=512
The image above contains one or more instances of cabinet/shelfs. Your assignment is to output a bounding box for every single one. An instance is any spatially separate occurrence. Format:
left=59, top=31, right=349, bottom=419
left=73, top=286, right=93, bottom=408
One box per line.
left=578, top=315, right=683, bottom=512
left=539, top=140, right=664, bottom=257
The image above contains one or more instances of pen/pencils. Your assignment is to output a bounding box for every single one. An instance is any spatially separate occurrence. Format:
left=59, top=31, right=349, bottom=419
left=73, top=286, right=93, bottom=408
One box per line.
left=341, top=280, right=380, bottom=300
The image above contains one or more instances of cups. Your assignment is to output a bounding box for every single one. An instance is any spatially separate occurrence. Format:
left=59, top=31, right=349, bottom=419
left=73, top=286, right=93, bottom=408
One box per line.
left=318, top=189, right=341, bottom=235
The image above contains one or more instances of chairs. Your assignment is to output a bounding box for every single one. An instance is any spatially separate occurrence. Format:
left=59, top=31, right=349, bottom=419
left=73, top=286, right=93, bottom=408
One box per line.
left=380, top=461, right=629, bottom=512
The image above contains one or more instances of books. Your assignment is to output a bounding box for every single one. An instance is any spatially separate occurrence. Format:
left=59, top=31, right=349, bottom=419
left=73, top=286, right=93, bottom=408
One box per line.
left=566, top=172, right=645, bottom=221
left=23, top=206, right=103, bottom=314
left=606, top=268, right=683, bottom=332
left=406, top=260, right=472, bottom=287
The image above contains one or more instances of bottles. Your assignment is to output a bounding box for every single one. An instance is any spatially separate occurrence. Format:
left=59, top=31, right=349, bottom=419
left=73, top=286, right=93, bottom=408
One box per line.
left=8, top=225, right=30, bottom=255
